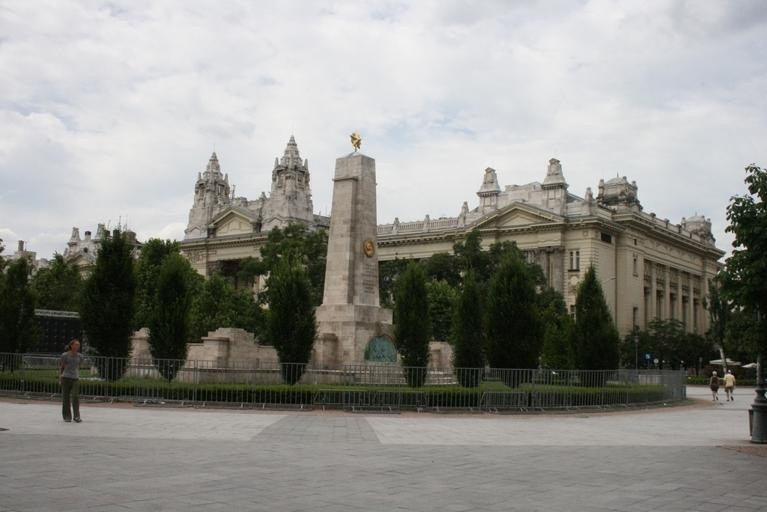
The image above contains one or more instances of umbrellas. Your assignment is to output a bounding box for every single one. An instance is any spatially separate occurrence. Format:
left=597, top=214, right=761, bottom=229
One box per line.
left=739, top=362, right=756, bottom=368
left=708, top=357, right=741, bottom=366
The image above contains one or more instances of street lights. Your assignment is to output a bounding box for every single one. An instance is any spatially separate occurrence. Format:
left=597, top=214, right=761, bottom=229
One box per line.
left=634, top=331, right=640, bottom=370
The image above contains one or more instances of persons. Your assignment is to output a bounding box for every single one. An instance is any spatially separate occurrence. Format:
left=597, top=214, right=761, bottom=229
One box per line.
left=722, top=369, right=735, bottom=401
left=708, top=370, right=719, bottom=400
left=58, top=339, right=83, bottom=423
